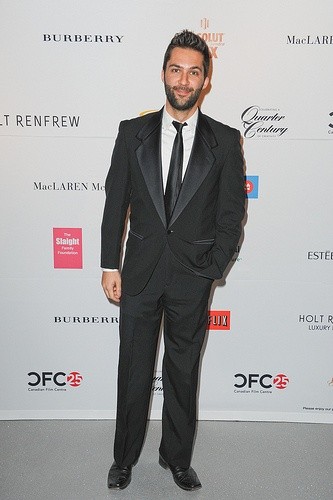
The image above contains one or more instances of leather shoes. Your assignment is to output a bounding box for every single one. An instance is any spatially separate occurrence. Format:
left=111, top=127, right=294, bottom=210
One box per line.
left=158, top=452, right=202, bottom=492
left=107, top=458, right=139, bottom=491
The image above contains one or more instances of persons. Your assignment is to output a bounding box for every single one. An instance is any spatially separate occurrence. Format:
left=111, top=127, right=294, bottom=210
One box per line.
left=99, top=29, right=245, bottom=490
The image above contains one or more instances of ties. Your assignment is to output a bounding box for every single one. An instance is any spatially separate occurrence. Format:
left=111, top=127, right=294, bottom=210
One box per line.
left=164, top=120, right=188, bottom=230
